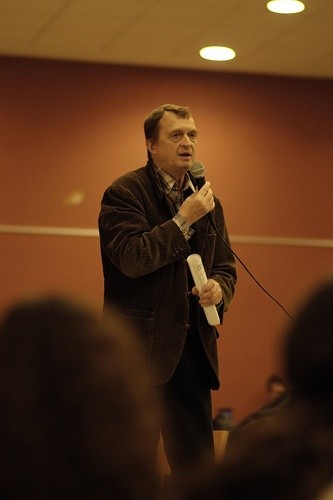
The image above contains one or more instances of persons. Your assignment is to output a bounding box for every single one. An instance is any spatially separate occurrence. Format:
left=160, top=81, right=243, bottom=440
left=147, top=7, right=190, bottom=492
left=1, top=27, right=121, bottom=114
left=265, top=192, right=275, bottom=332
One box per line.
left=191, top=402, right=333, bottom=500
left=95, top=102, right=238, bottom=468
left=1, top=293, right=165, bottom=500
left=264, top=376, right=283, bottom=401
left=280, top=278, right=333, bottom=404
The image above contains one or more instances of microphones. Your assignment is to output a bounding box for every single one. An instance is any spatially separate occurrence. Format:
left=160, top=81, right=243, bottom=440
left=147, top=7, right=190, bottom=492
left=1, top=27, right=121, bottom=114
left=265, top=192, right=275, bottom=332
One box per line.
left=190, top=161, right=216, bottom=230
